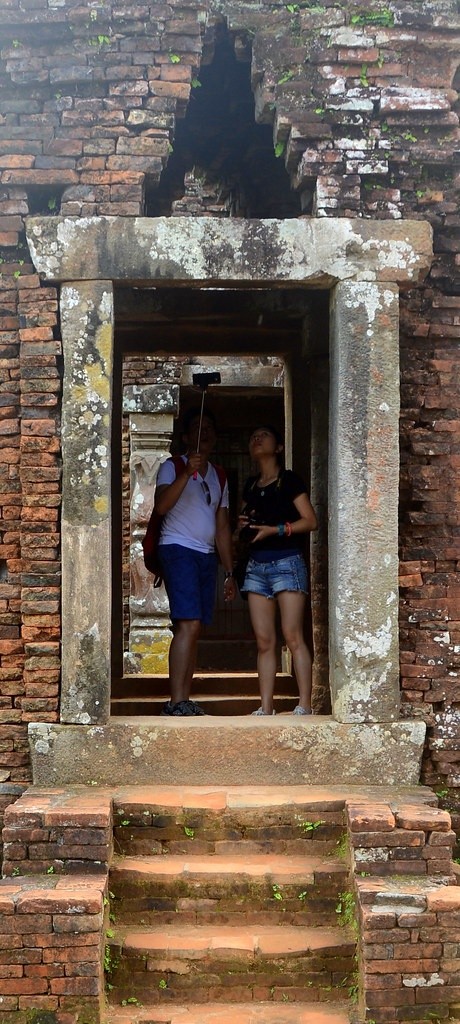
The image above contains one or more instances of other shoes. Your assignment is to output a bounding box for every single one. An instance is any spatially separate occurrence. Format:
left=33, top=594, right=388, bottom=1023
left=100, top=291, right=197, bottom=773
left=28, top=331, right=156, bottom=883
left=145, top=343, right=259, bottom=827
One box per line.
left=290, top=706, right=314, bottom=715
left=252, top=706, right=276, bottom=715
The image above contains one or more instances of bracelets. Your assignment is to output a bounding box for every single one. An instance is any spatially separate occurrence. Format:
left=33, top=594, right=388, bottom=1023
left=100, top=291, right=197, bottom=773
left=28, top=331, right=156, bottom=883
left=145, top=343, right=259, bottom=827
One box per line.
left=278, top=521, right=292, bottom=536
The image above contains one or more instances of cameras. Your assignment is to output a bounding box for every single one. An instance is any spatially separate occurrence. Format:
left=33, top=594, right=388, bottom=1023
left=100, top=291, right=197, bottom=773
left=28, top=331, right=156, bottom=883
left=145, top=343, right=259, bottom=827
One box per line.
left=239, top=516, right=266, bottom=542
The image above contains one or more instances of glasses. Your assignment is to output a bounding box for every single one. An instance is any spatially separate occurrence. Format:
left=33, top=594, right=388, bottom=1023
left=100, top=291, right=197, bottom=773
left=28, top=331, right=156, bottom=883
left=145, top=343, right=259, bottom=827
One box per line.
left=200, top=480, right=211, bottom=505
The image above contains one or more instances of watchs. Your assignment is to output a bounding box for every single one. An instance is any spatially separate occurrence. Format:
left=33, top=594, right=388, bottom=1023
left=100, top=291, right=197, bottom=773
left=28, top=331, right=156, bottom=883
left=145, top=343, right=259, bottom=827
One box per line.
left=224, top=571, right=233, bottom=578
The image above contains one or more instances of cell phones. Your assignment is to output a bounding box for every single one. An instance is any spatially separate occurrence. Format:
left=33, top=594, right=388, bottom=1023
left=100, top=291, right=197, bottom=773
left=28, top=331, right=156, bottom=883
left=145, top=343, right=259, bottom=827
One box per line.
left=193, top=372, right=221, bottom=385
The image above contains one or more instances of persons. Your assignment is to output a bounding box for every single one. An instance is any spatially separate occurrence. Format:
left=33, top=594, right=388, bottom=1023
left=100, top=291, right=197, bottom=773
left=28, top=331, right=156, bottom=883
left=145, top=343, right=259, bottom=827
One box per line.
left=230, top=425, right=319, bottom=717
left=152, top=411, right=236, bottom=717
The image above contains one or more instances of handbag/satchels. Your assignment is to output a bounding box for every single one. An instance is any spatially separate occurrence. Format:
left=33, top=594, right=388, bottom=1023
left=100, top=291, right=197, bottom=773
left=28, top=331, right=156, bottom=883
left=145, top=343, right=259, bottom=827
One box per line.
left=234, top=563, right=248, bottom=601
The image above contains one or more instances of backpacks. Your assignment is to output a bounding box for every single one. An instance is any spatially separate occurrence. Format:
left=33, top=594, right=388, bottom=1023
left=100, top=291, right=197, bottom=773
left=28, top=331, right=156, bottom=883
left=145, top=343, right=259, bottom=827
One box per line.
left=141, top=456, right=227, bottom=578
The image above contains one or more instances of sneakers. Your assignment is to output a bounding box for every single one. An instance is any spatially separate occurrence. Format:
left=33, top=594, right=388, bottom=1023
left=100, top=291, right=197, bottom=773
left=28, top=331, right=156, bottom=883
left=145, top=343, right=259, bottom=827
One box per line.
left=160, top=701, right=197, bottom=716
left=187, top=700, right=211, bottom=716
